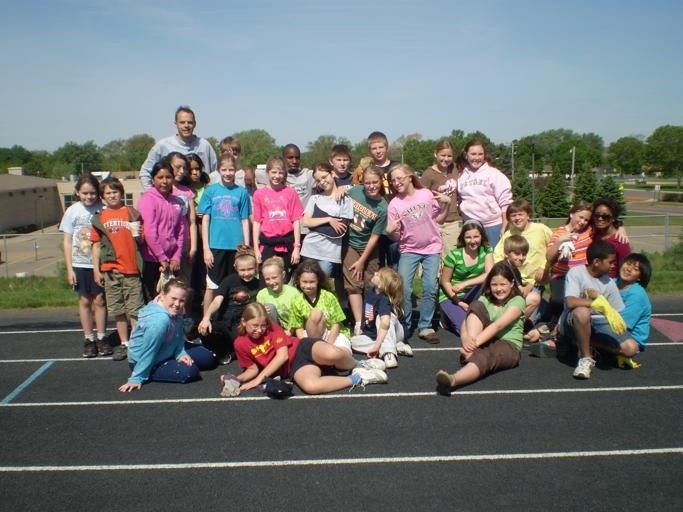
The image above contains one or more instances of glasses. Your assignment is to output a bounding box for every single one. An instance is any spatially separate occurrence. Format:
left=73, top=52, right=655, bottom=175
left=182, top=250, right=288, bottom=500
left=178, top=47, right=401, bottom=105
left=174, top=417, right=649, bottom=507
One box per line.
left=593, top=213, right=614, bottom=222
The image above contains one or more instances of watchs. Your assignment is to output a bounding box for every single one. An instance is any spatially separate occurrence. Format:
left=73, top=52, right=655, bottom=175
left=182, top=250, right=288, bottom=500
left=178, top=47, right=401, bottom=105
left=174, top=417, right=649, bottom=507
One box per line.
left=293, top=242, right=300, bottom=247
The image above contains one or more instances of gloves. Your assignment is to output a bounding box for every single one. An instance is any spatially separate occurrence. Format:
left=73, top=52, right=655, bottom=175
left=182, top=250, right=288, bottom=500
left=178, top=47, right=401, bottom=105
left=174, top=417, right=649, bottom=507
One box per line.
left=587, top=295, right=627, bottom=335
left=220, top=374, right=241, bottom=397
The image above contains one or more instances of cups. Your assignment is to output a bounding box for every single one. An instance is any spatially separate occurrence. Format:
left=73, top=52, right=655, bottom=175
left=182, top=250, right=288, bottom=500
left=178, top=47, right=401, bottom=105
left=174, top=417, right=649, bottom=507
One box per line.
left=129, top=222, right=140, bottom=237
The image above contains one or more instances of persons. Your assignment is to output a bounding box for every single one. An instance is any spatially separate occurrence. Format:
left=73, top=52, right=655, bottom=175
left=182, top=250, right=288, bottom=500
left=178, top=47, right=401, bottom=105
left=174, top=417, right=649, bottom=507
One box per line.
left=483, top=236, right=541, bottom=343
left=546, top=202, right=629, bottom=306
left=207, top=136, right=257, bottom=217
left=167, top=152, right=197, bottom=286
left=351, top=131, right=400, bottom=267
left=590, top=198, right=631, bottom=278
left=420, top=141, right=464, bottom=278
left=242, top=144, right=317, bottom=244
left=289, top=258, right=353, bottom=376
left=185, top=153, right=209, bottom=209
left=140, top=106, right=217, bottom=188
left=457, top=137, right=513, bottom=249
left=197, top=244, right=263, bottom=366
left=255, top=255, right=325, bottom=341
left=559, top=239, right=624, bottom=378
left=137, top=159, right=186, bottom=299
left=437, top=220, right=495, bottom=337
left=221, top=303, right=389, bottom=394
left=252, top=155, right=303, bottom=283
left=387, top=162, right=452, bottom=343
left=300, top=161, right=353, bottom=281
left=495, top=200, right=553, bottom=343
left=60, top=174, right=114, bottom=358
left=91, top=177, right=144, bottom=361
left=118, top=278, right=217, bottom=393
left=353, top=267, right=413, bottom=369
left=575, top=253, right=651, bottom=370
left=329, top=145, right=355, bottom=185
left=336, top=166, right=388, bottom=335
left=199, top=153, right=252, bottom=316
left=435, top=262, right=526, bottom=396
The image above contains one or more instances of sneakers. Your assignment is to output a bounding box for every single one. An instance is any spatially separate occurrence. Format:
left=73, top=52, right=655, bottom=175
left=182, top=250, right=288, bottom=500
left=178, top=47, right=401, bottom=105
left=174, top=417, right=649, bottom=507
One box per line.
left=81, top=340, right=96, bottom=359
left=96, top=337, right=112, bottom=355
left=572, top=357, right=595, bottom=380
left=112, top=345, right=126, bottom=360
left=351, top=367, right=388, bottom=387
left=360, top=359, right=385, bottom=371
left=396, top=341, right=413, bottom=357
left=434, top=370, right=454, bottom=395
left=418, top=328, right=440, bottom=344
left=384, top=353, right=397, bottom=367
